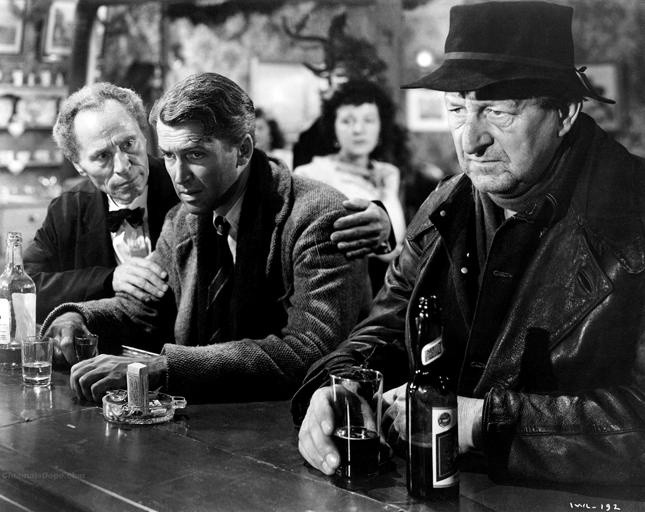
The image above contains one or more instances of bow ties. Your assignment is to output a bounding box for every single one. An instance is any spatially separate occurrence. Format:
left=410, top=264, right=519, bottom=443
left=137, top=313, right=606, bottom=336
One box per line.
left=102, top=207, right=146, bottom=233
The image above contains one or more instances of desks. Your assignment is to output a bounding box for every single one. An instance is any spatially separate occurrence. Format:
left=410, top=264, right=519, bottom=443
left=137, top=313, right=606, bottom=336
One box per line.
left=0, top=345, right=645, bottom=512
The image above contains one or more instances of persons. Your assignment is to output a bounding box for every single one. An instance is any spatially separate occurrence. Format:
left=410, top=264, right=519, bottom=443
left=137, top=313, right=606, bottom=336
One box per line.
left=290, top=34, right=418, bottom=223
left=29, top=72, right=373, bottom=401
left=253, top=105, right=292, bottom=173
left=291, top=6, right=645, bottom=498
left=291, top=79, right=408, bottom=264
left=4, top=82, right=397, bottom=353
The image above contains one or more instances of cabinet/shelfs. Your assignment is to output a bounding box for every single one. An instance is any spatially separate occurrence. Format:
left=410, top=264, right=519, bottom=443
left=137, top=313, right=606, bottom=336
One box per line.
left=1, top=0, right=108, bottom=271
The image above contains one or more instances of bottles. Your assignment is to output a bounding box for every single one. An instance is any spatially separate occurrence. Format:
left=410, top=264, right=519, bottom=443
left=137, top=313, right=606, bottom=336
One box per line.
left=1, top=231, right=38, bottom=370
left=405, top=297, right=461, bottom=501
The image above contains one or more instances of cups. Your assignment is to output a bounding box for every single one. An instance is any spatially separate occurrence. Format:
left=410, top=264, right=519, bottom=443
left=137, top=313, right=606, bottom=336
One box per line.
left=19, top=334, right=54, bottom=386
left=74, top=335, right=99, bottom=360
left=328, top=368, right=383, bottom=486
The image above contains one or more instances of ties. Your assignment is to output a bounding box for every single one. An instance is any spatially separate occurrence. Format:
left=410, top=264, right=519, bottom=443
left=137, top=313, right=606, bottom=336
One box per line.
left=204, top=218, right=237, bottom=346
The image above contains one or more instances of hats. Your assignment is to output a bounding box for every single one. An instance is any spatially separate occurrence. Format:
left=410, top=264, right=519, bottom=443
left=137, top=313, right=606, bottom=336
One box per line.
left=397, top=0, right=620, bottom=107
